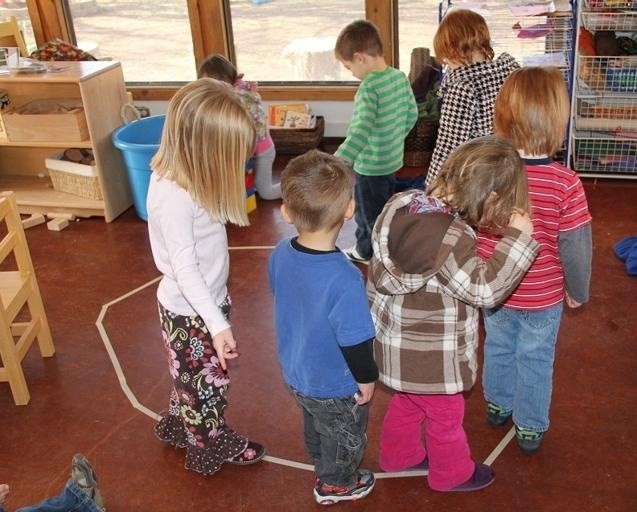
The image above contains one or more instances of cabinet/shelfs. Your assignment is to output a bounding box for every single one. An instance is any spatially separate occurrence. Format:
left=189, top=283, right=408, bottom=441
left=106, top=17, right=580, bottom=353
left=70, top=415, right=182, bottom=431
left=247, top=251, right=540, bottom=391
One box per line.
left=439, top=0, right=576, bottom=171
left=0, top=59, right=137, bottom=224
left=565, top=0, right=637, bottom=180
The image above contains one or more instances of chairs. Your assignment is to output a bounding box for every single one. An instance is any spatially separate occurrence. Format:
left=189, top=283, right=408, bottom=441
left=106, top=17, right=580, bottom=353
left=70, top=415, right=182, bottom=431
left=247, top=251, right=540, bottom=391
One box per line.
left=0, top=16, right=28, bottom=60
left=0, top=189, right=58, bottom=407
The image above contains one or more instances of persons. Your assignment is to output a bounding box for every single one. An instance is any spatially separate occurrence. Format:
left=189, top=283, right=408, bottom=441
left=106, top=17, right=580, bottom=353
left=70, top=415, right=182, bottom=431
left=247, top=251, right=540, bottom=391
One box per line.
left=196, top=52, right=282, bottom=201
left=422, top=6, right=522, bottom=187
left=0, top=451, right=106, bottom=511
left=332, top=19, right=419, bottom=261
left=145, top=77, right=267, bottom=476
left=267, top=149, right=379, bottom=506
left=457, top=65, right=595, bottom=456
left=364, top=133, right=542, bottom=494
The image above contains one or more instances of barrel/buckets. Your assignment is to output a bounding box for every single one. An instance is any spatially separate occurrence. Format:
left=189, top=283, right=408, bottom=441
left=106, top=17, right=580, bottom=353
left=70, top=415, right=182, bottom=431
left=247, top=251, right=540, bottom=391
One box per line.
left=111, top=113, right=168, bottom=223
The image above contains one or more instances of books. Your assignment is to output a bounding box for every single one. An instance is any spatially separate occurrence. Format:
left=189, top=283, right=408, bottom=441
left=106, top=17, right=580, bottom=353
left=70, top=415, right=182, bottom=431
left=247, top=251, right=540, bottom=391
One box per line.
left=265, top=102, right=316, bottom=128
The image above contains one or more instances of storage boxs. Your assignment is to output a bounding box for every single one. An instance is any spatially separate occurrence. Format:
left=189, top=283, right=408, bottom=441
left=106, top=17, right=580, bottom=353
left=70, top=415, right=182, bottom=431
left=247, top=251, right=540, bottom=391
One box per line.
left=3, top=98, right=91, bottom=145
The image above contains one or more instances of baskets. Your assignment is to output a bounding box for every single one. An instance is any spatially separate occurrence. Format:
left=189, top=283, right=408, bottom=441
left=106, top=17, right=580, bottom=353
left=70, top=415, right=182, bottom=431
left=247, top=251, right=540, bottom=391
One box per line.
left=44, top=149, right=102, bottom=201
left=404, top=120, right=439, bottom=167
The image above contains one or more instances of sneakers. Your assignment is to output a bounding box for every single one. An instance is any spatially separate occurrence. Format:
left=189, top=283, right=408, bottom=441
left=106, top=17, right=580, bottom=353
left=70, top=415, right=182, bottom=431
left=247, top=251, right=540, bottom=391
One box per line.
left=312, top=468, right=375, bottom=506
left=485, top=402, right=512, bottom=426
left=514, top=424, right=543, bottom=452
left=231, top=440, right=266, bottom=465
left=341, top=244, right=369, bottom=266
left=70, top=453, right=106, bottom=512
left=447, top=463, right=496, bottom=491
left=409, top=457, right=428, bottom=470
